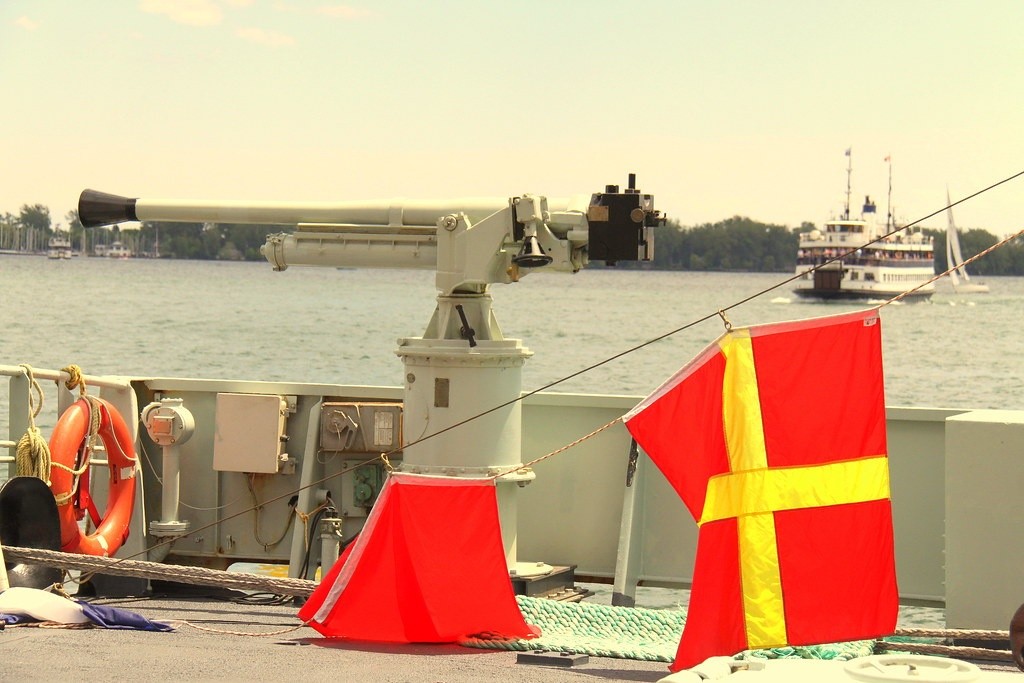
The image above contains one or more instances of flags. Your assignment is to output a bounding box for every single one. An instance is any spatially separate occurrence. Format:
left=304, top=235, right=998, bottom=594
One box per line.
left=294, top=470, right=539, bottom=644
left=845, top=149, right=851, bottom=156
left=884, top=156, right=890, bottom=161
left=622, top=308, right=900, bottom=675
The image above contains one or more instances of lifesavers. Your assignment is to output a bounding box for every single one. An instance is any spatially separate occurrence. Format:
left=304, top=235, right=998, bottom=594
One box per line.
left=44, top=393, right=139, bottom=558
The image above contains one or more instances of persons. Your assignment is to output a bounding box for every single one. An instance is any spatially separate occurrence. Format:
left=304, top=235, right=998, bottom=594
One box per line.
left=790, top=248, right=934, bottom=265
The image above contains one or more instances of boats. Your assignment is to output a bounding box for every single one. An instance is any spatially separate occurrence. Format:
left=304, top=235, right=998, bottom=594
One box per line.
left=792, top=147, right=935, bottom=298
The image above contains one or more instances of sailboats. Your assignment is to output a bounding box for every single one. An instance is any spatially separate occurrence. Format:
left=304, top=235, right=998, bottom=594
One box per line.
left=942, top=184, right=989, bottom=294
